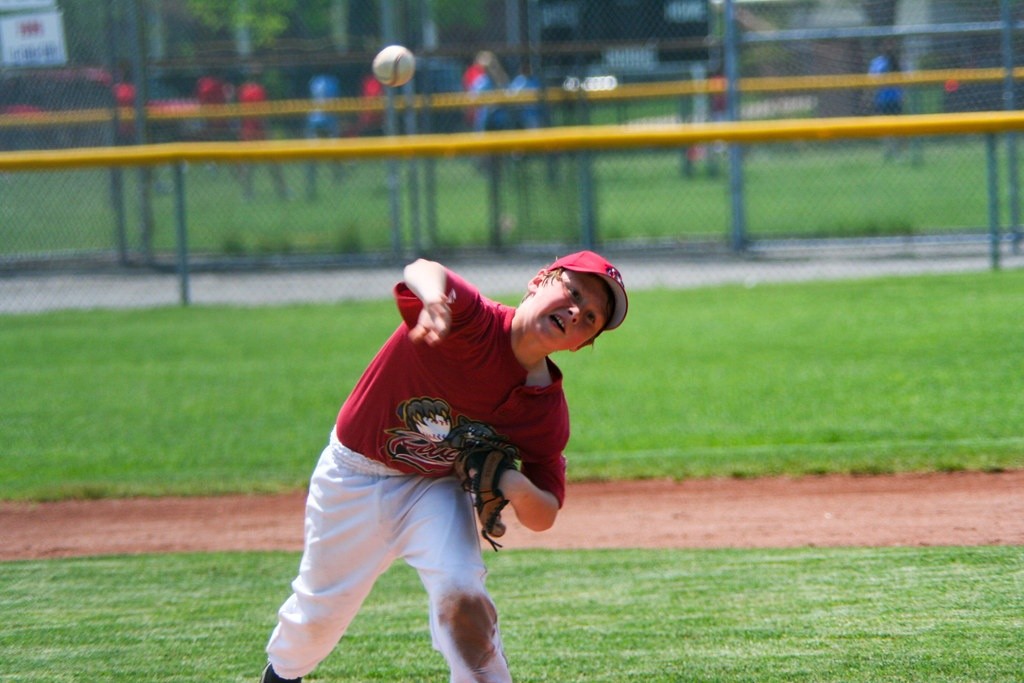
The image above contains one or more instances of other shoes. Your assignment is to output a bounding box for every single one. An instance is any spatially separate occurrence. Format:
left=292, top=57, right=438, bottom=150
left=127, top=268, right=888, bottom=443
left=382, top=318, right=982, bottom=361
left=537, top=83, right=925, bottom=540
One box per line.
left=258, top=657, right=303, bottom=683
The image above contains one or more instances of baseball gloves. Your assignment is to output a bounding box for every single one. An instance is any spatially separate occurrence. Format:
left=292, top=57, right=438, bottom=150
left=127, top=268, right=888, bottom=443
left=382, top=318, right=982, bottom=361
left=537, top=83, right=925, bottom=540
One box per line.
left=453, top=435, right=523, bottom=554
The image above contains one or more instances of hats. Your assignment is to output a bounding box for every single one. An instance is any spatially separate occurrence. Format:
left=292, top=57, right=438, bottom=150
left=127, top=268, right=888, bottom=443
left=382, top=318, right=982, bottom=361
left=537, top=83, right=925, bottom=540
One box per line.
left=546, top=250, right=629, bottom=330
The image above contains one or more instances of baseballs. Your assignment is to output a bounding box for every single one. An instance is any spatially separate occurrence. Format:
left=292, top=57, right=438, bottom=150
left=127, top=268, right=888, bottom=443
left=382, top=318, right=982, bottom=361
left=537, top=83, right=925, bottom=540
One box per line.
left=372, top=43, right=417, bottom=88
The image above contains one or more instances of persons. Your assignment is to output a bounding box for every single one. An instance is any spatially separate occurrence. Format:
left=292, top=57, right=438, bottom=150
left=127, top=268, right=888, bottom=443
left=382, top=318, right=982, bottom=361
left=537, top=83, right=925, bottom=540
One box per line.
left=869, top=37, right=905, bottom=159
left=707, top=59, right=728, bottom=157
left=259, top=250, right=629, bottom=683
left=112, top=49, right=563, bottom=209
